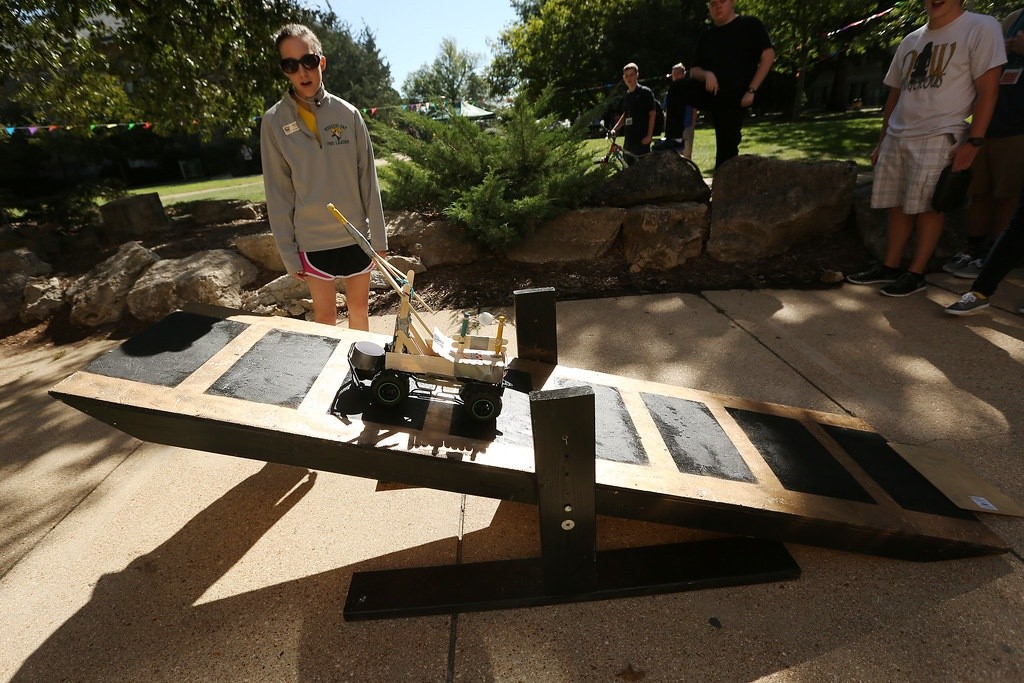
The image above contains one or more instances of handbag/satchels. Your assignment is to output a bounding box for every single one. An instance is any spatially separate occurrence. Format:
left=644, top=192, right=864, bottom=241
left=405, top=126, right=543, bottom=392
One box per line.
left=932, top=165, right=968, bottom=214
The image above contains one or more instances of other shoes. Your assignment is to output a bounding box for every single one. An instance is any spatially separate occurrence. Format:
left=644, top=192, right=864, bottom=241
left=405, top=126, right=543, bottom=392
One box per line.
left=652, top=137, right=684, bottom=153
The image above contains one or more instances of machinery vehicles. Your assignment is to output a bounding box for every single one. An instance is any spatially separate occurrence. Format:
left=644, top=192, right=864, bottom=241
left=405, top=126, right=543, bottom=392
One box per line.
left=325, top=202, right=513, bottom=425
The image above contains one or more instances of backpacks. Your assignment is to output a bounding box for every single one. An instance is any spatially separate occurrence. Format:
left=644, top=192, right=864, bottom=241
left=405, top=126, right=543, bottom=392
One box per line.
left=626, top=84, right=664, bottom=136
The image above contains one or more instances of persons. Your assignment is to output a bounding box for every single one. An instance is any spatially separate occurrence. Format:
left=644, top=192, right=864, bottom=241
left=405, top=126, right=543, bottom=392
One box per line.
left=652, top=1, right=775, bottom=170
left=846, top=1, right=1024, bottom=315
left=258, top=23, right=389, bottom=331
left=609, top=63, right=656, bottom=167
left=663, top=62, right=700, bottom=163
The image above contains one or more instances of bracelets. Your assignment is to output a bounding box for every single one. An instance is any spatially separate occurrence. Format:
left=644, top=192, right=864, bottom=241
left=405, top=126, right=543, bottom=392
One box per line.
left=747, top=89, right=755, bottom=94
left=966, top=137, right=985, bottom=146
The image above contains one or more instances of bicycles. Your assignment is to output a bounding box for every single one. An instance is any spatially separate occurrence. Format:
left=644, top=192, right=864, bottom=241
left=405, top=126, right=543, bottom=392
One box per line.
left=579, top=119, right=703, bottom=185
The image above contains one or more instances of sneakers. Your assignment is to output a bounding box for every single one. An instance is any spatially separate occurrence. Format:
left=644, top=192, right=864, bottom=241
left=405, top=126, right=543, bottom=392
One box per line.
left=953, top=258, right=984, bottom=279
left=944, top=292, right=990, bottom=315
left=846, top=262, right=901, bottom=285
left=942, top=249, right=974, bottom=273
left=879, top=270, right=927, bottom=297
left=1019, top=308, right=1024, bottom=313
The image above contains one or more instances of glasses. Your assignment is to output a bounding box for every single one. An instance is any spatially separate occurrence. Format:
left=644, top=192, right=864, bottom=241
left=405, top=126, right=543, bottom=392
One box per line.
left=281, top=54, right=320, bottom=74
